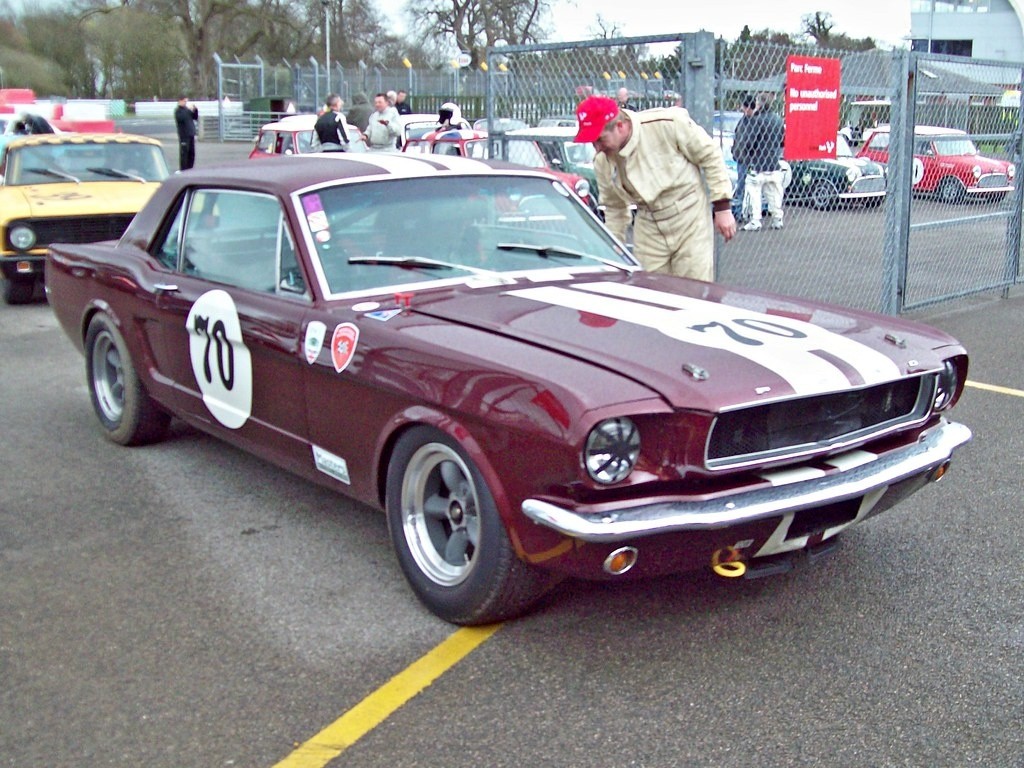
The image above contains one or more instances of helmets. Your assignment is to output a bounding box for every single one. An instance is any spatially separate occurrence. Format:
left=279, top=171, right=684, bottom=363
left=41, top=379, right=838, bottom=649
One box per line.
left=435, top=102, right=461, bottom=126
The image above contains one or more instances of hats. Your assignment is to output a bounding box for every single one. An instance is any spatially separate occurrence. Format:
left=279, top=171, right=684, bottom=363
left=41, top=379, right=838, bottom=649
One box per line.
left=574, top=94, right=620, bottom=144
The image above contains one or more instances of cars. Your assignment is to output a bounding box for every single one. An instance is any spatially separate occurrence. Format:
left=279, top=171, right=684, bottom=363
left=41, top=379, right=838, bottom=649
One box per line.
left=399, top=129, right=592, bottom=239
left=778, top=133, right=888, bottom=209
left=857, top=125, right=1016, bottom=205
left=575, top=86, right=678, bottom=100
left=395, top=111, right=745, bottom=158
left=0, top=125, right=220, bottom=303
left=249, top=113, right=372, bottom=157
left=42, top=149, right=976, bottom=626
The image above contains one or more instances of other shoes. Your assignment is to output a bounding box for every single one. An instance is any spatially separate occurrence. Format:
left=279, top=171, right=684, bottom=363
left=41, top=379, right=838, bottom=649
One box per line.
left=771, top=220, right=785, bottom=228
left=739, top=220, right=761, bottom=231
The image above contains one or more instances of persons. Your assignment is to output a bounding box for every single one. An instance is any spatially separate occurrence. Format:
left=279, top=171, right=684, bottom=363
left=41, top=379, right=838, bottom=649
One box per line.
left=437, top=102, right=465, bottom=130
left=357, top=93, right=401, bottom=151
left=616, top=88, right=636, bottom=111
left=312, top=94, right=351, bottom=153
left=386, top=90, right=397, bottom=107
left=12, top=113, right=55, bottom=135
left=396, top=90, right=413, bottom=115
left=347, top=90, right=373, bottom=133
left=576, top=97, right=736, bottom=284
left=731, top=93, right=786, bottom=231
left=174, top=94, right=198, bottom=171
